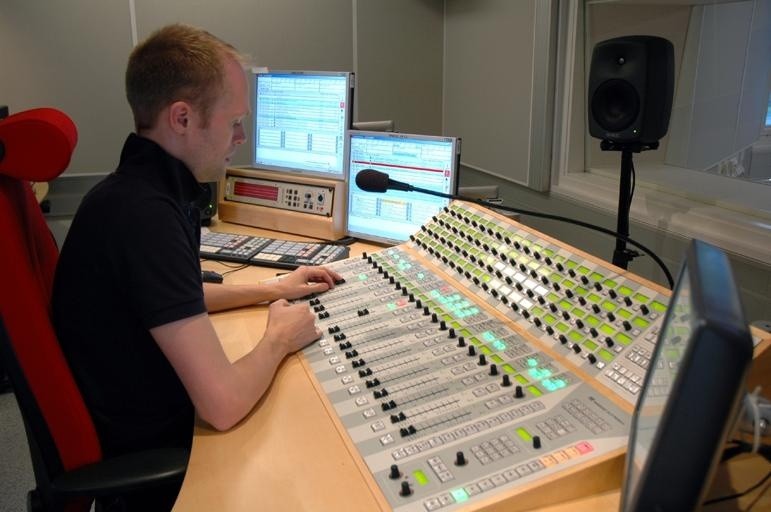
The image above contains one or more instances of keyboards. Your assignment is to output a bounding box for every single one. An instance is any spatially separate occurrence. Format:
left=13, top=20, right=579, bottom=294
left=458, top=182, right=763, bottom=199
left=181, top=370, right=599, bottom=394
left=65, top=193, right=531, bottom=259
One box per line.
left=199, top=229, right=347, bottom=270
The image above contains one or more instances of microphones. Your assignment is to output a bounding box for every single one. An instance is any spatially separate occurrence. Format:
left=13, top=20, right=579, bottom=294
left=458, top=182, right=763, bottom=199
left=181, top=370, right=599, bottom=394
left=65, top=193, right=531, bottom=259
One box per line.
left=355, top=169, right=673, bottom=287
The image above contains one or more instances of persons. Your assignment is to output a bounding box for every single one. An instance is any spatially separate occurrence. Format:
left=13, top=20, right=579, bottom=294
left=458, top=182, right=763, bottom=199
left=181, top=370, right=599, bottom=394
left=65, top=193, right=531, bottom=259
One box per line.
left=46, top=20, right=342, bottom=511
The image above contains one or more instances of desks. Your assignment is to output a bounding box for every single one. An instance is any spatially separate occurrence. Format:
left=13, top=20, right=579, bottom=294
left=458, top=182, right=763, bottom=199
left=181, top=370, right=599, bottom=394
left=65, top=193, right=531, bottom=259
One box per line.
left=172, top=197, right=771, bottom=512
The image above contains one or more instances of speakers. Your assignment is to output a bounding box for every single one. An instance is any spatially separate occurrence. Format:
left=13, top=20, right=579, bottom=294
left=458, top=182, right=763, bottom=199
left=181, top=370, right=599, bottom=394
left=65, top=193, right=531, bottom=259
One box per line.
left=586, top=34, right=674, bottom=152
left=198, top=181, right=218, bottom=227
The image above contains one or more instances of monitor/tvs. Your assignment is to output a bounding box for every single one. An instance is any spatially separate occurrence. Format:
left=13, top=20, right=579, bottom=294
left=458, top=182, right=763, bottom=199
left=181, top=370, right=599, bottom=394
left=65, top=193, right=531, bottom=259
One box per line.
left=343, top=128, right=462, bottom=247
left=249, top=69, right=354, bottom=181
left=618, top=238, right=754, bottom=512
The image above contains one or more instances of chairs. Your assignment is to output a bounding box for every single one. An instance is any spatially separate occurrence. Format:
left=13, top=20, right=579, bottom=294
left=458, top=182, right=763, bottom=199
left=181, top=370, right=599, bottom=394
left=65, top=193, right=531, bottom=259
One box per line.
left=0, top=105, right=189, bottom=512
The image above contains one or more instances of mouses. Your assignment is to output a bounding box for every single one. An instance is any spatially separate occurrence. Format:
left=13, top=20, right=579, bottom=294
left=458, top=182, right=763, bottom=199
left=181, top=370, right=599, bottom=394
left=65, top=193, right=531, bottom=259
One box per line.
left=201, top=270, right=223, bottom=284
